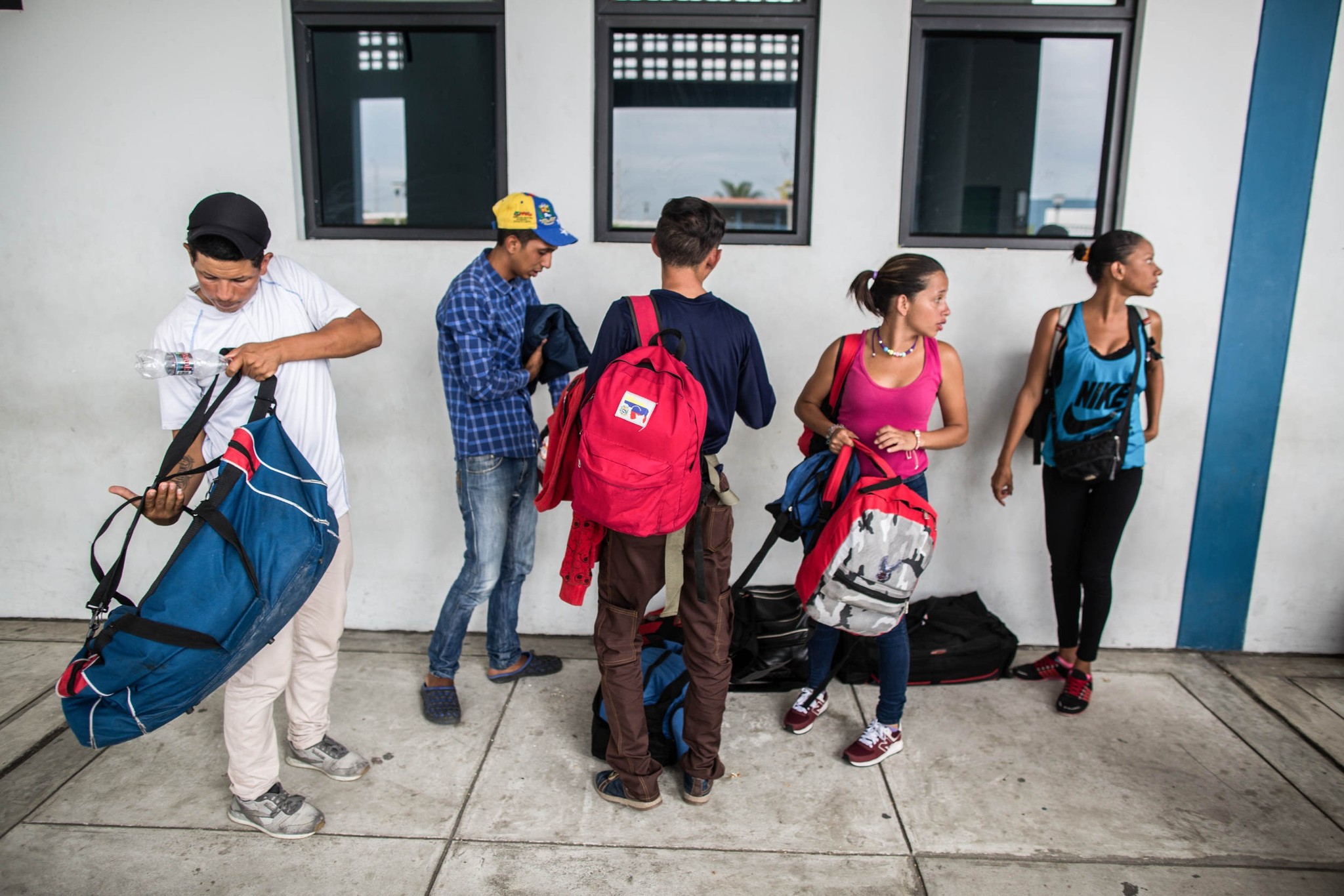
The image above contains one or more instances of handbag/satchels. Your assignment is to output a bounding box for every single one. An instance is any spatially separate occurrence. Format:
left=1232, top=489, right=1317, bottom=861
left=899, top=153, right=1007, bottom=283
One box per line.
left=590, top=638, right=691, bottom=768
left=1053, top=427, right=1128, bottom=482
left=797, top=334, right=862, bottom=456
left=729, top=448, right=855, bottom=693
left=637, top=607, right=683, bottom=648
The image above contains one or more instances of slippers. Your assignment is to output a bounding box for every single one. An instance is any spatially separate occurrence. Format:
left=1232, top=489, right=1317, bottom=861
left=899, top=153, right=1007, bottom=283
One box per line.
left=487, top=650, right=563, bottom=684
left=421, top=683, right=461, bottom=725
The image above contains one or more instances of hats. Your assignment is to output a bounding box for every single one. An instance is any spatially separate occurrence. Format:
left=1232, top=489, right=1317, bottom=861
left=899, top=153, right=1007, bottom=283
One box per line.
left=490, top=193, right=579, bottom=247
left=187, top=192, right=271, bottom=262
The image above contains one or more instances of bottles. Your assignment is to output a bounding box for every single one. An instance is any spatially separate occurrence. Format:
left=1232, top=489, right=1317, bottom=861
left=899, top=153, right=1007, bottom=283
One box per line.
left=134, top=348, right=235, bottom=379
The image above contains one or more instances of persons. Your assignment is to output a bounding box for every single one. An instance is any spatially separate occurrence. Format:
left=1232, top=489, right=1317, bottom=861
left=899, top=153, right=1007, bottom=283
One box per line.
left=109, top=192, right=391, bottom=840
left=991, top=230, right=1163, bottom=716
left=584, top=196, right=777, bottom=810
left=782, top=253, right=969, bottom=768
left=419, top=190, right=571, bottom=723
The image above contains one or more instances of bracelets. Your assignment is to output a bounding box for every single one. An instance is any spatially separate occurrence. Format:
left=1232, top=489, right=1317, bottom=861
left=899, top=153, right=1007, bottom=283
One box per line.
left=826, top=423, right=844, bottom=439
left=907, top=429, right=921, bottom=470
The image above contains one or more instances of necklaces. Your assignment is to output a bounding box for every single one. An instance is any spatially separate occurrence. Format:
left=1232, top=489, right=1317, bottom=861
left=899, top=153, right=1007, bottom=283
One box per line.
left=871, top=325, right=919, bottom=357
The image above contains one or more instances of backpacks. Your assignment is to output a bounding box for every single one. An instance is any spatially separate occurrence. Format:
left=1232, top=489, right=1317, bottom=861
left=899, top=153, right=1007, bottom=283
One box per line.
left=572, top=296, right=707, bottom=537
left=53, top=348, right=339, bottom=749
left=833, top=591, right=1020, bottom=687
left=794, top=437, right=938, bottom=637
left=1021, top=302, right=1143, bottom=441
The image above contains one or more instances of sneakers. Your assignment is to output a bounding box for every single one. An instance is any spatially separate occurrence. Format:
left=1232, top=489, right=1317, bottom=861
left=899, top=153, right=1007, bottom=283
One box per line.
left=682, top=771, right=715, bottom=804
left=228, top=781, right=326, bottom=840
left=284, top=734, right=370, bottom=782
left=1011, top=650, right=1076, bottom=681
left=842, top=721, right=906, bottom=768
left=594, top=770, right=663, bottom=811
left=1056, top=667, right=1093, bottom=714
left=784, top=686, right=830, bottom=732
